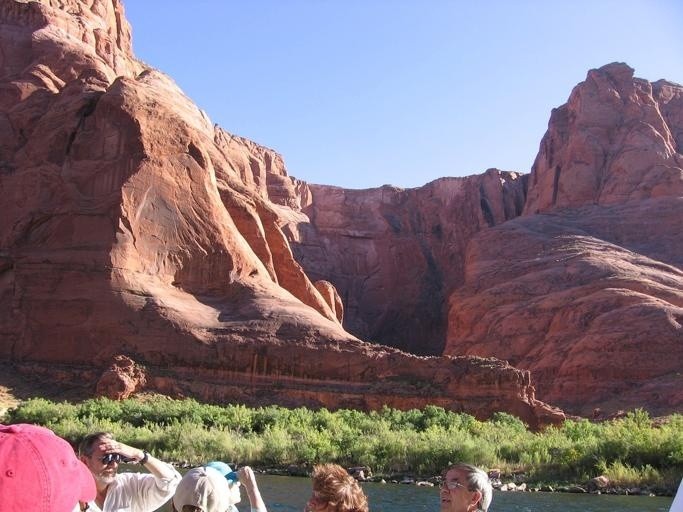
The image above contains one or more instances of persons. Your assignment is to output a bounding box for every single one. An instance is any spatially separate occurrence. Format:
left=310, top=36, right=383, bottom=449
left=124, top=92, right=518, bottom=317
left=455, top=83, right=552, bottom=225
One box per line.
left=439, top=463, right=493, bottom=512
left=172, top=466, right=231, bottom=512
left=302, top=463, right=369, bottom=512
left=204, top=461, right=268, bottom=512
left=74, top=430, right=182, bottom=512
left=0, top=420, right=98, bottom=512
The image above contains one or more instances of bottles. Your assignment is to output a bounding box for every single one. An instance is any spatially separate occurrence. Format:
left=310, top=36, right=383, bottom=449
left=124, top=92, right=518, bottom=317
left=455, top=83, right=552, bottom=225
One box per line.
left=173, top=466, right=230, bottom=512
left=204, top=462, right=239, bottom=485
left=0, top=423, right=97, bottom=512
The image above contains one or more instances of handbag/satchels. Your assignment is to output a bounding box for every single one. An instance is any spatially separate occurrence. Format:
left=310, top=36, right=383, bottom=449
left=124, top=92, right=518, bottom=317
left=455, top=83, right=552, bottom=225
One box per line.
left=139, top=449, right=148, bottom=466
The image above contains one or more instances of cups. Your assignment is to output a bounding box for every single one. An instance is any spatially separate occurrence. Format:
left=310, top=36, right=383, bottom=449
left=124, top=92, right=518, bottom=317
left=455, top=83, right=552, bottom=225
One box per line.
left=439, top=481, right=468, bottom=490
left=96, top=454, right=121, bottom=464
